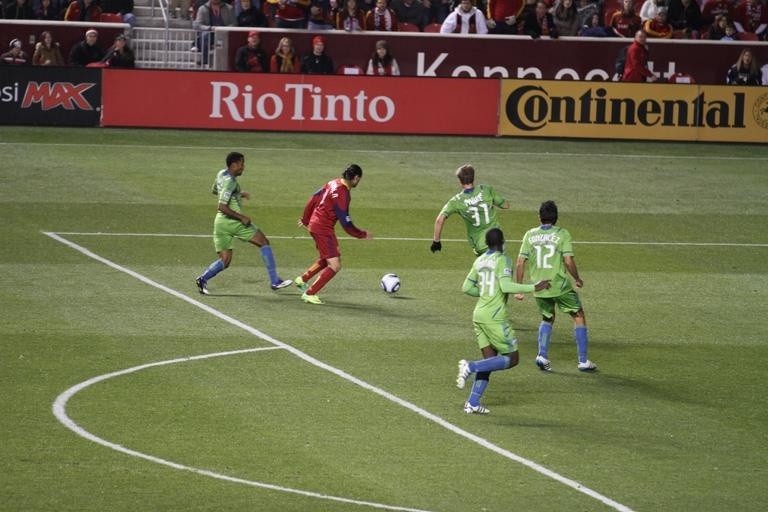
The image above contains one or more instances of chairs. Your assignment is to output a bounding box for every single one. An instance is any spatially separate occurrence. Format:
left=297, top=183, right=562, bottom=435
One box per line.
left=195, top=276, right=210, bottom=295
left=578, top=359, right=597, bottom=371
left=271, top=279, right=294, bottom=290
left=456, top=358, right=472, bottom=389
left=535, top=354, right=551, bottom=371
left=464, top=401, right=490, bottom=414
left=301, top=290, right=322, bottom=305
left=294, top=276, right=309, bottom=293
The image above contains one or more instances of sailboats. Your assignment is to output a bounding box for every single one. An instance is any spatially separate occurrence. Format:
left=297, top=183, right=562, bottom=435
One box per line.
left=380, top=273, right=401, bottom=292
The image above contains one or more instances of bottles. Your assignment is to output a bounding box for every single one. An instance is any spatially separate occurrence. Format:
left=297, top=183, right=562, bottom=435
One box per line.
left=112, top=32, right=126, bottom=41
left=312, top=36, right=328, bottom=44
left=248, top=32, right=261, bottom=38
left=375, top=40, right=388, bottom=50
left=85, top=29, right=99, bottom=38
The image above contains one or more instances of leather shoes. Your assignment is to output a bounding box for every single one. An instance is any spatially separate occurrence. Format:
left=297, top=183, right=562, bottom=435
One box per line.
left=431, top=240, right=441, bottom=253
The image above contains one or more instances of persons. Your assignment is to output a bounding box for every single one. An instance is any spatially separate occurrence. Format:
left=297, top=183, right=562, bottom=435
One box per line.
left=513, top=200, right=598, bottom=370
left=301, top=35, right=334, bottom=74
left=69, top=29, right=105, bottom=66
left=702, top=13, right=732, bottom=39
left=641, top=6, right=674, bottom=39
left=366, top=0, right=399, bottom=31
left=440, top=0, right=488, bottom=34
left=238, top=0, right=271, bottom=28
left=294, top=164, right=373, bottom=305
left=456, top=228, right=552, bottom=414
left=441, top=1, right=462, bottom=24
left=579, top=13, right=610, bottom=37
left=640, top=0, right=670, bottom=26
left=195, top=151, right=293, bottom=295
left=0, top=38, right=30, bottom=65
left=308, top=0, right=345, bottom=30
left=699, top=0, right=730, bottom=23
left=196, top=0, right=238, bottom=70
left=63, top=0, right=103, bottom=22
left=486, top=0, right=527, bottom=35
left=726, top=48, right=762, bottom=85
left=336, top=0, right=366, bottom=31
left=36, top=0, right=62, bottom=20
left=547, top=0, right=580, bottom=36
left=430, top=164, right=511, bottom=259
left=620, top=30, right=658, bottom=83
left=720, top=23, right=737, bottom=40
left=191, top=20, right=201, bottom=53
left=523, top=2, right=559, bottom=42
left=4, top=0, right=38, bottom=19
left=32, top=31, right=68, bottom=66
left=101, top=33, right=136, bottom=66
left=609, top=0, right=642, bottom=37
left=399, top=0, right=440, bottom=32
left=269, top=37, right=301, bottom=73
left=580, top=0, right=622, bottom=12
left=93, top=0, right=135, bottom=28
left=666, top=0, right=708, bottom=31
left=169, top=0, right=192, bottom=20
left=730, top=0, right=768, bottom=40
left=266, top=0, right=312, bottom=30
left=235, top=31, right=270, bottom=73
left=366, top=40, right=400, bottom=76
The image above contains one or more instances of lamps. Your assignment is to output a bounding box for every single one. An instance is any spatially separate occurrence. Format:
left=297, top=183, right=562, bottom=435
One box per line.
left=737, top=31, right=761, bottom=41
left=667, top=73, right=696, bottom=83
left=334, top=64, right=363, bottom=74
left=400, top=22, right=420, bottom=31
left=602, top=0, right=644, bottom=24
left=99, top=12, right=123, bottom=21
left=670, top=27, right=699, bottom=39
left=423, top=23, right=441, bottom=32
